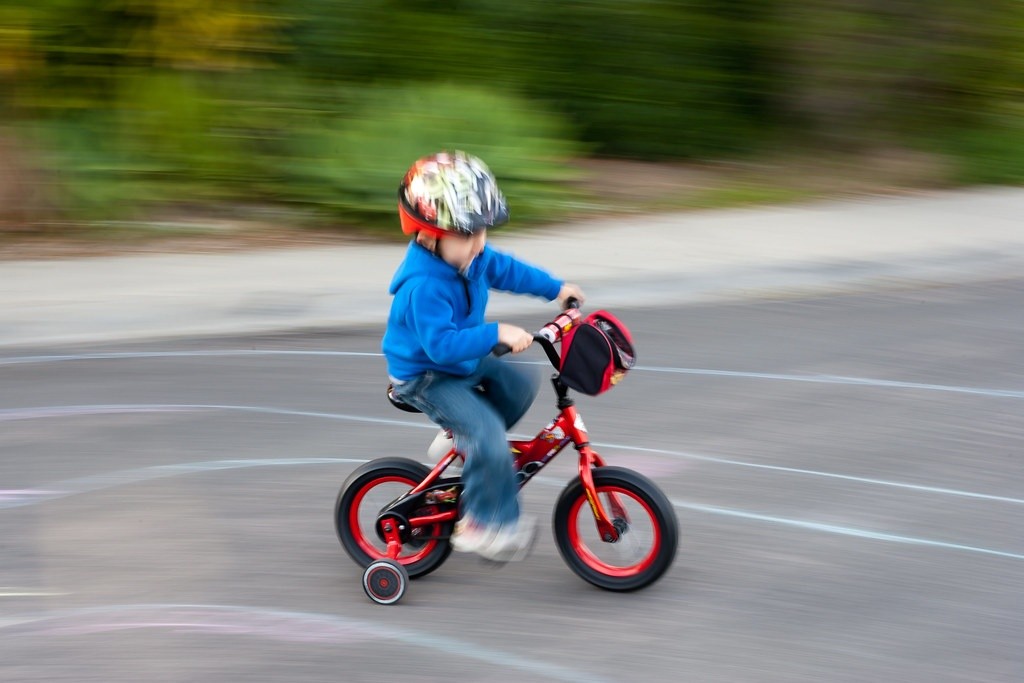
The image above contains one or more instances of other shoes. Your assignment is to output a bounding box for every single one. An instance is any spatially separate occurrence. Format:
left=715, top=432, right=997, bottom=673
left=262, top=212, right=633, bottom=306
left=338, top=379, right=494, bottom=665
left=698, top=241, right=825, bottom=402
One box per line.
left=449, top=517, right=534, bottom=551
left=428, top=426, right=465, bottom=467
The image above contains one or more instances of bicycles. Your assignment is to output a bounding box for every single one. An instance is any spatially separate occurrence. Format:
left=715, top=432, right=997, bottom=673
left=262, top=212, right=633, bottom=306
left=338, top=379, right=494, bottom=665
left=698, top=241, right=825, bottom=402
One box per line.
left=330, top=293, right=681, bottom=607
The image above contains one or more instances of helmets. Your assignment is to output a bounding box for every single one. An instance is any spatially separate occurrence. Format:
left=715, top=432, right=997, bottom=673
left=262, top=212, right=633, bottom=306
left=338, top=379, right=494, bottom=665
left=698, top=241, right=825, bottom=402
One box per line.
left=398, top=149, right=508, bottom=237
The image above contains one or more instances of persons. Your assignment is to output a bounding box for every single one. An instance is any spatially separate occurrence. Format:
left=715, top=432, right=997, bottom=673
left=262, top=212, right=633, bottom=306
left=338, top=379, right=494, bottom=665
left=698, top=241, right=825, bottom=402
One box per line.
left=381, top=150, right=585, bottom=564
